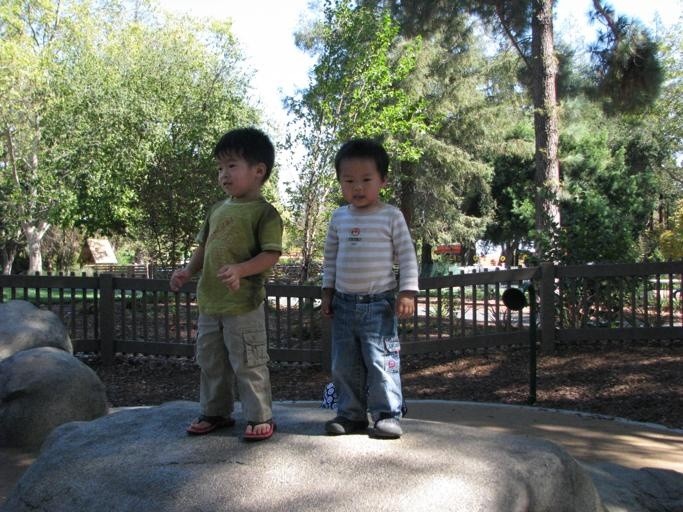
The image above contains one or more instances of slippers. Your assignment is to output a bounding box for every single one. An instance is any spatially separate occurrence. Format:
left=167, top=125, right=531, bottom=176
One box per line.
left=241, top=418, right=277, bottom=440
left=187, top=414, right=235, bottom=435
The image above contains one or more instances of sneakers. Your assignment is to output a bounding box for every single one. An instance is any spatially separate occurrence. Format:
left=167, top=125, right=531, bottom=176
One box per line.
left=325, top=415, right=368, bottom=434
left=369, top=416, right=403, bottom=437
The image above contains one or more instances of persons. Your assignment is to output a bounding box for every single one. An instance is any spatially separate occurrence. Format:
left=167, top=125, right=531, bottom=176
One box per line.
left=167, top=125, right=283, bottom=442
left=316, top=137, right=419, bottom=438
